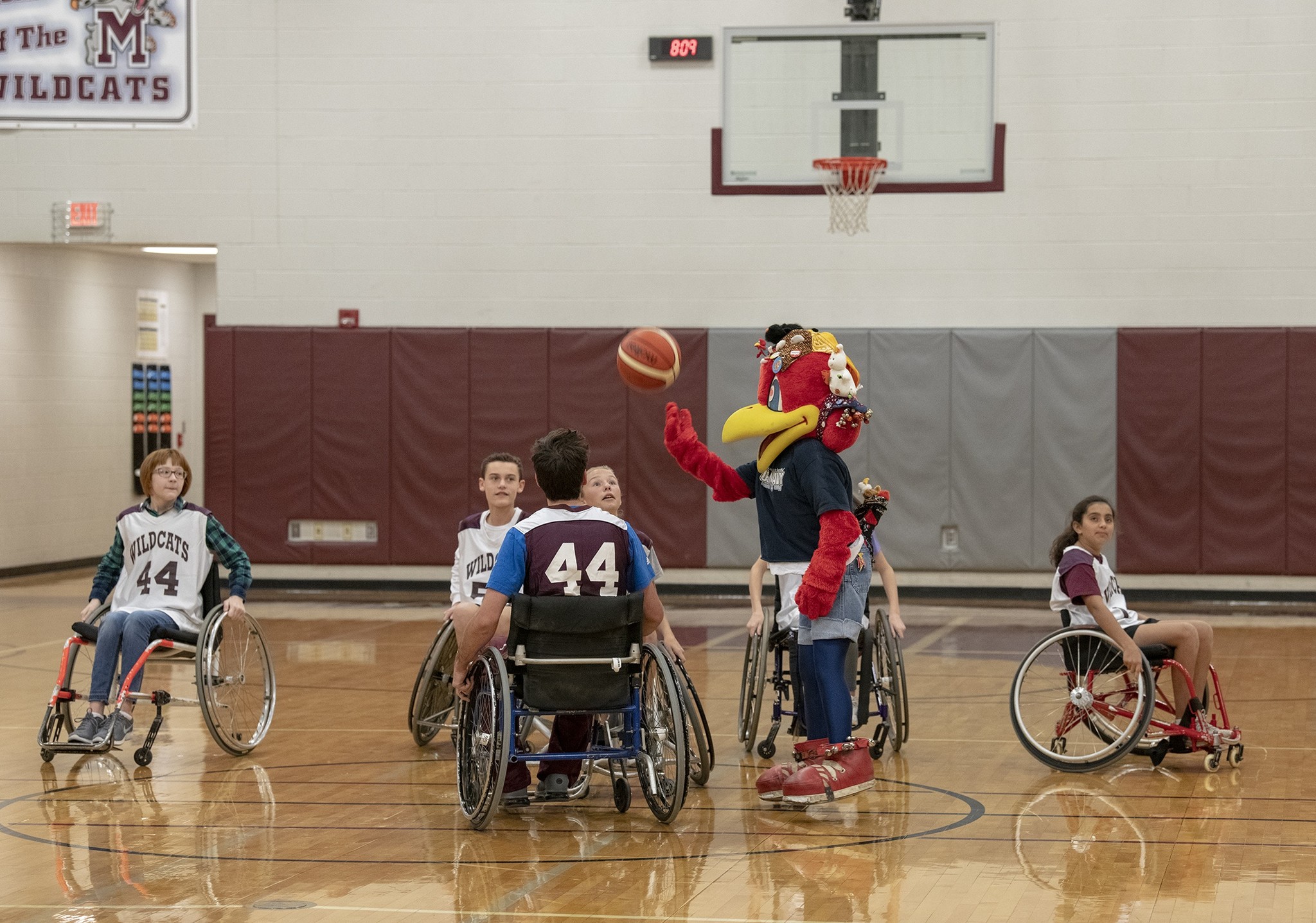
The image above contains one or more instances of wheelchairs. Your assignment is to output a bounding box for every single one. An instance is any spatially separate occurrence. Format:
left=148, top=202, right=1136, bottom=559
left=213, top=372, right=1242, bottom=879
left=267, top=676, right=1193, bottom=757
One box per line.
left=738, top=572, right=910, bottom=760
left=1010, top=608, right=1245, bottom=775
left=455, top=594, right=692, bottom=834
left=37, top=560, right=278, bottom=766
left=409, top=605, right=718, bottom=786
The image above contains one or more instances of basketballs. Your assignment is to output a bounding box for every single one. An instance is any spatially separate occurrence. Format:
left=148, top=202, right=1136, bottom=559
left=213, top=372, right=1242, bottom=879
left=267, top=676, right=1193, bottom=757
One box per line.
left=617, top=327, right=682, bottom=394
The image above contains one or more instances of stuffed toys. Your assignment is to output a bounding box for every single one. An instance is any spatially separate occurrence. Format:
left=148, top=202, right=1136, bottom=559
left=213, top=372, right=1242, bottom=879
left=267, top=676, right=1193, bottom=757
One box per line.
left=663, top=324, right=889, bottom=806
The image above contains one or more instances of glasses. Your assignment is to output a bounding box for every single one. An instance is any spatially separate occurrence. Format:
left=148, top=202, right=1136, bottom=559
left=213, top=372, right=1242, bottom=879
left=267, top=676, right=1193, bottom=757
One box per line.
left=152, top=467, right=188, bottom=479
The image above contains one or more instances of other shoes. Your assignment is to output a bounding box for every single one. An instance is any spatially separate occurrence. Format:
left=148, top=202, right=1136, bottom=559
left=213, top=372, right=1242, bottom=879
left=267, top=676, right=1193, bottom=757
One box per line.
left=534, top=774, right=571, bottom=801
left=1195, top=709, right=1237, bottom=741
left=850, top=694, right=858, bottom=726
left=486, top=788, right=531, bottom=806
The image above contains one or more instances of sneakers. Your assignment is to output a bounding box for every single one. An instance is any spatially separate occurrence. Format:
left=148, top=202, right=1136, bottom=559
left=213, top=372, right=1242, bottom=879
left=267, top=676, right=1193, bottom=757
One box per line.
left=68, top=707, right=106, bottom=743
left=93, top=706, right=135, bottom=747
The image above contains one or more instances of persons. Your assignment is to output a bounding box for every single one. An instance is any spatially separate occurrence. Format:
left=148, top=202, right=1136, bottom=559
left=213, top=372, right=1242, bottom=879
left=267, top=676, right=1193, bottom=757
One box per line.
left=443, top=428, right=907, bottom=809
left=1047, top=497, right=1240, bottom=750
left=65, top=448, right=252, bottom=748
